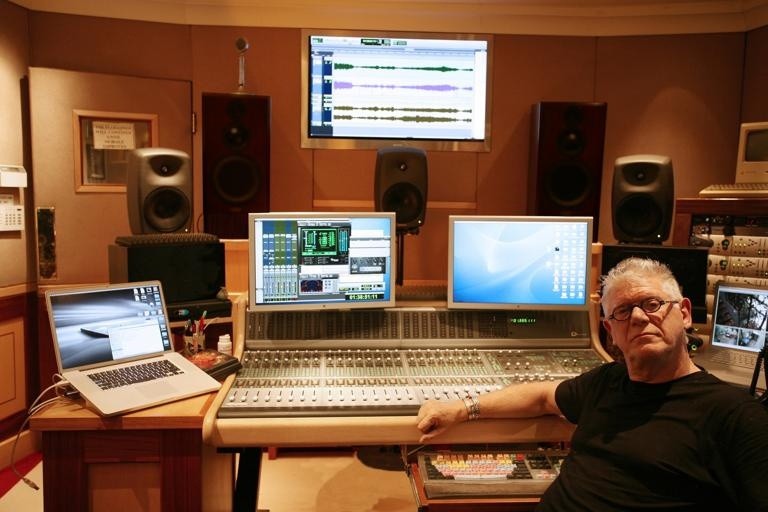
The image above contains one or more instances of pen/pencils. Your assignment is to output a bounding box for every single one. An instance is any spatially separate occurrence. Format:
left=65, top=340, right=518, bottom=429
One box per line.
left=184, top=310, right=207, bottom=336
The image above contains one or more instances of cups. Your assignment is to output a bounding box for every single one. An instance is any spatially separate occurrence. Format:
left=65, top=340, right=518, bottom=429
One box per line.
left=183, top=333, right=206, bottom=358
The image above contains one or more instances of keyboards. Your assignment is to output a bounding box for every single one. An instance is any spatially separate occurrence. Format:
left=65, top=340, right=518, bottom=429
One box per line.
left=114, top=232, right=220, bottom=247
left=699, top=183, right=768, bottom=198
left=416, top=449, right=569, bottom=499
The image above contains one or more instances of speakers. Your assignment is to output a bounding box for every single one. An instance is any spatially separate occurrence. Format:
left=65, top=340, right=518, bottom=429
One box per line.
left=127, top=146, right=194, bottom=235
left=202, top=92, right=270, bottom=239
left=527, top=101, right=608, bottom=242
left=610, top=154, right=675, bottom=243
left=600, top=244, right=709, bottom=324
left=374, top=146, right=428, bottom=235
left=107, top=243, right=225, bottom=303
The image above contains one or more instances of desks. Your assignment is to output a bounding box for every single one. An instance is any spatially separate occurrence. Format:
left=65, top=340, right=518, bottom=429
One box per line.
left=199, top=236, right=708, bottom=512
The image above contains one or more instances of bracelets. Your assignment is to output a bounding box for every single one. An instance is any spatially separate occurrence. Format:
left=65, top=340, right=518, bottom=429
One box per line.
left=462, top=396, right=481, bottom=421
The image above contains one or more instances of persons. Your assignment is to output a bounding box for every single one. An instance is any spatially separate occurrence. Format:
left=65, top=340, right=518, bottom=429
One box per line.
left=417, top=257, right=767, bottom=510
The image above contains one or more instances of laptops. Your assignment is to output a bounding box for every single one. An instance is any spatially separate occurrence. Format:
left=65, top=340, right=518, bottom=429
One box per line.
left=45, top=280, right=223, bottom=418
left=692, top=282, right=768, bottom=390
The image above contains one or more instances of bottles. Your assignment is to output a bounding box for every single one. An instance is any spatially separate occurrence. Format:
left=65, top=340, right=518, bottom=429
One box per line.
left=218, top=335, right=232, bottom=356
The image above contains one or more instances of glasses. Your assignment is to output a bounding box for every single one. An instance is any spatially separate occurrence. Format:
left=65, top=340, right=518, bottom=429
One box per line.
left=608, top=296, right=679, bottom=321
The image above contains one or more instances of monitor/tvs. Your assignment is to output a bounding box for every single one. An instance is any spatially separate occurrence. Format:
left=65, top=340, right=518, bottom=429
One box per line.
left=298, top=28, right=494, bottom=154
left=247, top=212, right=396, bottom=313
left=735, top=122, right=768, bottom=183
left=447, top=214, right=594, bottom=311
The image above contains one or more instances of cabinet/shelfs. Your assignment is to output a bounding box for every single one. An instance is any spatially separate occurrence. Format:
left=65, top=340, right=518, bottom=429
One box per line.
left=30, top=342, right=235, bottom=511
